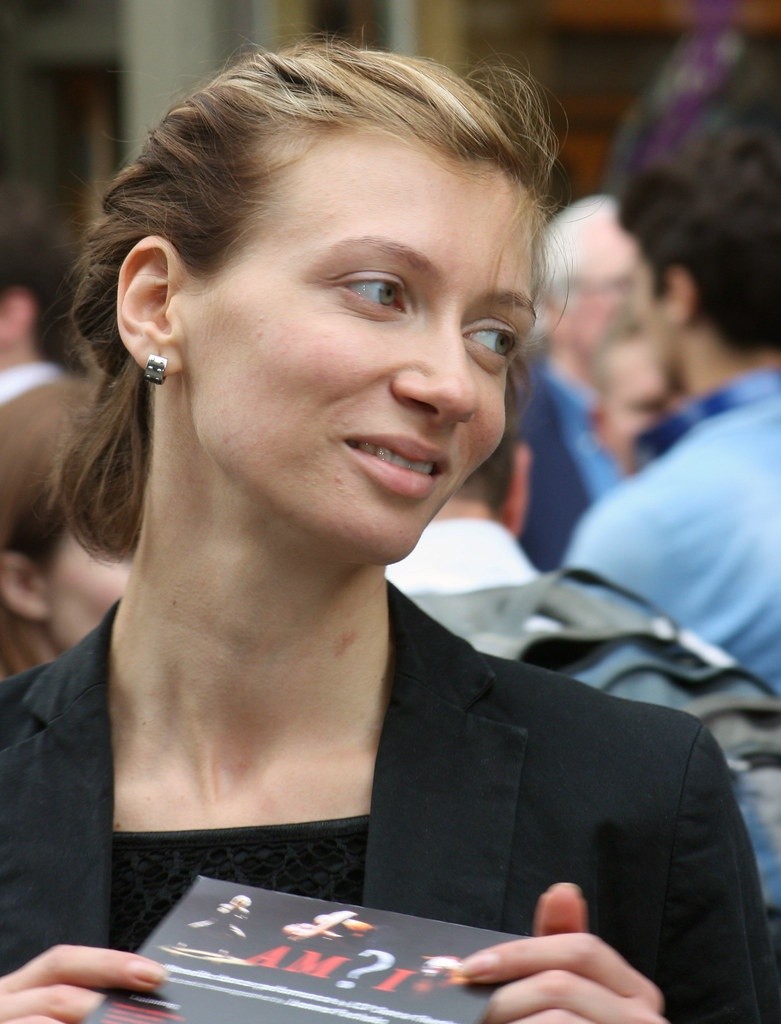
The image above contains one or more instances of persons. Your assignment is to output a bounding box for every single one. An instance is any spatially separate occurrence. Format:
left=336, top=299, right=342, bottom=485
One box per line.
left=0, top=21, right=781, bottom=1024
